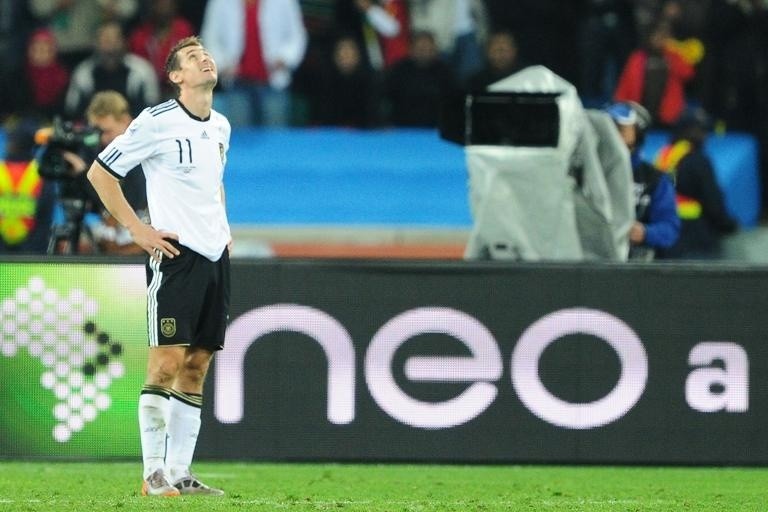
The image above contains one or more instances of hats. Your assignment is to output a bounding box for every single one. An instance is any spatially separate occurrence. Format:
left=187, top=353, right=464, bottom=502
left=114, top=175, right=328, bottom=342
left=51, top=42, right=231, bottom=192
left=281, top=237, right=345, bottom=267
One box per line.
left=601, top=100, right=651, bottom=132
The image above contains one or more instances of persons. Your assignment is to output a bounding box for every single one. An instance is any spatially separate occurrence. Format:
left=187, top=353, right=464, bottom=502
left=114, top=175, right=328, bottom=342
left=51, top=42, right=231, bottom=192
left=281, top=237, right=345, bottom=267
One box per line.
left=2, top=1, right=768, bottom=161
left=81, top=92, right=150, bottom=254
left=86, top=37, right=231, bottom=499
left=603, top=104, right=741, bottom=265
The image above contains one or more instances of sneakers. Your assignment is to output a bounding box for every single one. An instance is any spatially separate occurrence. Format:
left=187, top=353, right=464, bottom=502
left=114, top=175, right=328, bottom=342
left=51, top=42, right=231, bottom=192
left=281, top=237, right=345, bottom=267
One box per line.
left=174, top=478, right=223, bottom=496
left=140, top=470, right=181, bottom=497
